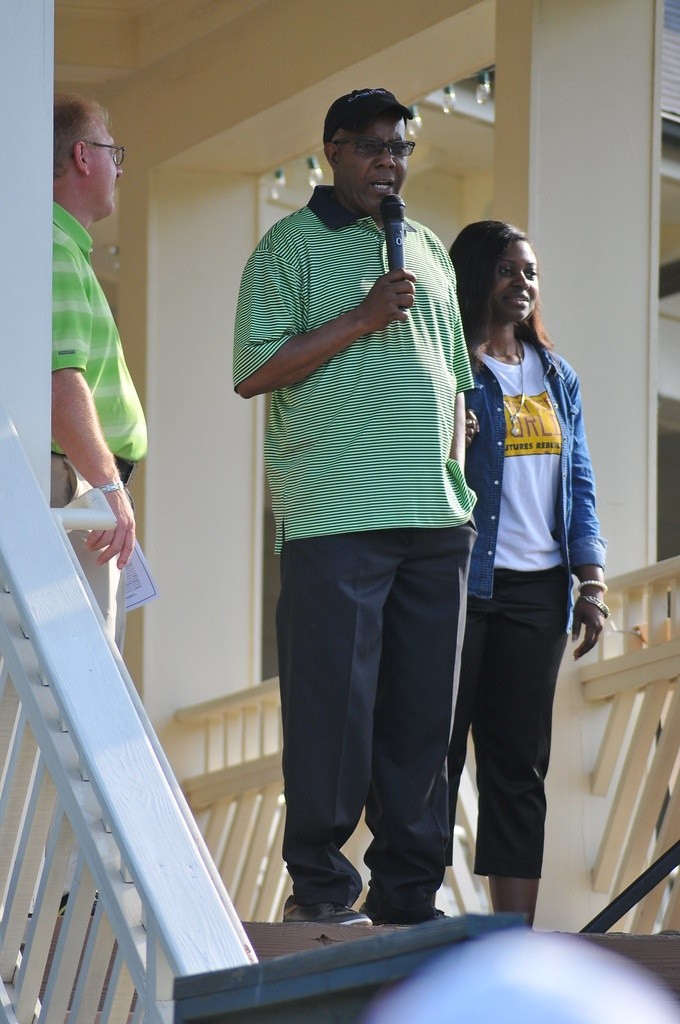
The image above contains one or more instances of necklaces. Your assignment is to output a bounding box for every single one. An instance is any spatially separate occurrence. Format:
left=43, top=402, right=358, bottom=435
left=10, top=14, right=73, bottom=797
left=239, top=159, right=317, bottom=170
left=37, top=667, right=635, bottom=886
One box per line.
left=503, top=342, right=525, bottom=436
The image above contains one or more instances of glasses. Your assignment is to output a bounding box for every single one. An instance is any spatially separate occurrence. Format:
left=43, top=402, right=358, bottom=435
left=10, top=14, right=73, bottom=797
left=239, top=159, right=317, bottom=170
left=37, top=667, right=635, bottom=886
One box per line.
left=71, top=141, right=126, bottom=165
left=334, top=137, right=416, bottom=157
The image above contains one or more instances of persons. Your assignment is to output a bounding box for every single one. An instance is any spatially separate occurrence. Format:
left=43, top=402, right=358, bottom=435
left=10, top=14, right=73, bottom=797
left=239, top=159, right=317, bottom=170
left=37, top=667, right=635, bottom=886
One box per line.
left=232, top=86, right=476, bottom=925
left=50, top=97, right=148, bottom=914
left=450, top=219, right=610, bottom=927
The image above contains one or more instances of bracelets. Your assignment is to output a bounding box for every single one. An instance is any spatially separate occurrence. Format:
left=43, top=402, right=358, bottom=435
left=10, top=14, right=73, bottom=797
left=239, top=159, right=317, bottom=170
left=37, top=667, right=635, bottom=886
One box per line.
left=94, top=481, right=124, bottom=494
left=578, top=580, right=607, bottom=593
left=583, top=596, right=610, bottom=619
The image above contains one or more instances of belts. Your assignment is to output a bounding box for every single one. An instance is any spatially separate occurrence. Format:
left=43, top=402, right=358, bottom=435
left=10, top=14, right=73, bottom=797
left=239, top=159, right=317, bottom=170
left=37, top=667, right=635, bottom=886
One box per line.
left=114, top=451, right=133, bottom=483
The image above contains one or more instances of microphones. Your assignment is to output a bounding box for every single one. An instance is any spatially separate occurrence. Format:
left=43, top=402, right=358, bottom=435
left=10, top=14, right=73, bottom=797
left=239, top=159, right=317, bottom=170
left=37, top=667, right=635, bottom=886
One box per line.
left=380, top=194, right=406, bottom=311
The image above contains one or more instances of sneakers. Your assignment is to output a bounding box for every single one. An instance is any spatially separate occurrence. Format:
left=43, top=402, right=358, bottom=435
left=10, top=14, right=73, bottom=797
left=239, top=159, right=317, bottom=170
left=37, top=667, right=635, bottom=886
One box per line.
left=282, top=895, right=374, bottom=926
left=358, top=901, right=445, bottom=925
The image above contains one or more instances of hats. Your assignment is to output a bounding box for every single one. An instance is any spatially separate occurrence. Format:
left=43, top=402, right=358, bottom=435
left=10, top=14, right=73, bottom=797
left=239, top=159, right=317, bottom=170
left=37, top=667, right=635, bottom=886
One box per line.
left=323, top=87, right=413, bottom=145
